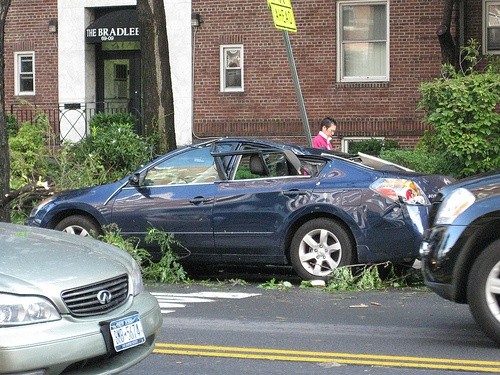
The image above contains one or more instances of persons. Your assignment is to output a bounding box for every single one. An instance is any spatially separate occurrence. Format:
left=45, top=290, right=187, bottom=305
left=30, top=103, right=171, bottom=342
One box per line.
left=312, top=116, right=337, bottom=151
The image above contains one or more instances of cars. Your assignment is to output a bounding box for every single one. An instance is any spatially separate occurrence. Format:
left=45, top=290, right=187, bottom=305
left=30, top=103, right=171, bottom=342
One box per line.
left=0, top=222, right=162, bottom=375
left=419, top=171, right=500, bottom=344
left=25, top=137, right=458, bottom=280
